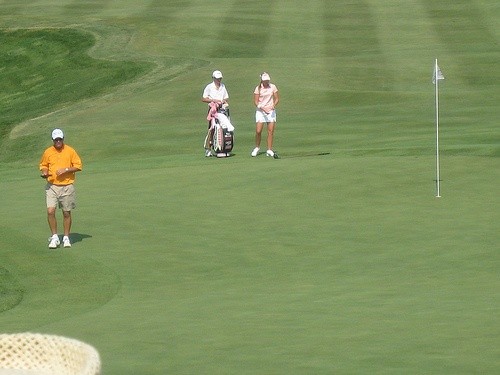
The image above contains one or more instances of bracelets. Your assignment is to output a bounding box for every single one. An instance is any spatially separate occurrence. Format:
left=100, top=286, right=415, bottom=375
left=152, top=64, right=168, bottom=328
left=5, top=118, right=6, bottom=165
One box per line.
left=66, top=168, right=68, bottom=173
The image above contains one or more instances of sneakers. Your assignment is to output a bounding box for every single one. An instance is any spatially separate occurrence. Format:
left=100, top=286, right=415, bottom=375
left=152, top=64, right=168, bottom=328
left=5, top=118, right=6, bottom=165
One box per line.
left=266, top=149, right=275, bottom=157
left=217, top=153, right=229, bottom=157
left=205, top=150, right=213, bottom=157
left=251, top=147, right=261, bottom=157
left=63, top=235, right=71, bottom=248
left=47, top=235, right=60, bottom=248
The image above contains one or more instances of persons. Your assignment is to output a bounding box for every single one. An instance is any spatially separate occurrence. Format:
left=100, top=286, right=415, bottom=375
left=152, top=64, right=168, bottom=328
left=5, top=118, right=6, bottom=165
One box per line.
left=202, top=71, right=228, bottom=157
left=251, top=73, right=280, bottom=156
left=40, top=129, right=82, bottom=248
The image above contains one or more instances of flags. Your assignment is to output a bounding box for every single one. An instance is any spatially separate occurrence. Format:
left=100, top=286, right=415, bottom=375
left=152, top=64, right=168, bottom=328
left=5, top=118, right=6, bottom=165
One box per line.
left=432, top=64, right=444, bottom=85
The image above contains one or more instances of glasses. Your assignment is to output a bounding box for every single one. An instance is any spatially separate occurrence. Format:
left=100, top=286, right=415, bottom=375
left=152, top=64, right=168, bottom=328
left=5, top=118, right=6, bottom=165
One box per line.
left=52, top=138, right=63, bottom=141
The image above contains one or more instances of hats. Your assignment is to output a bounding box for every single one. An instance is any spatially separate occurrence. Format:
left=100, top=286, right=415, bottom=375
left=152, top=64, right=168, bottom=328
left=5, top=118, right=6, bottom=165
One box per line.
left=51, top=129, right=64, bottom=140
left=260, top=74, right=270, bottom=80
left=212, top=70, right=223, bottom=78
